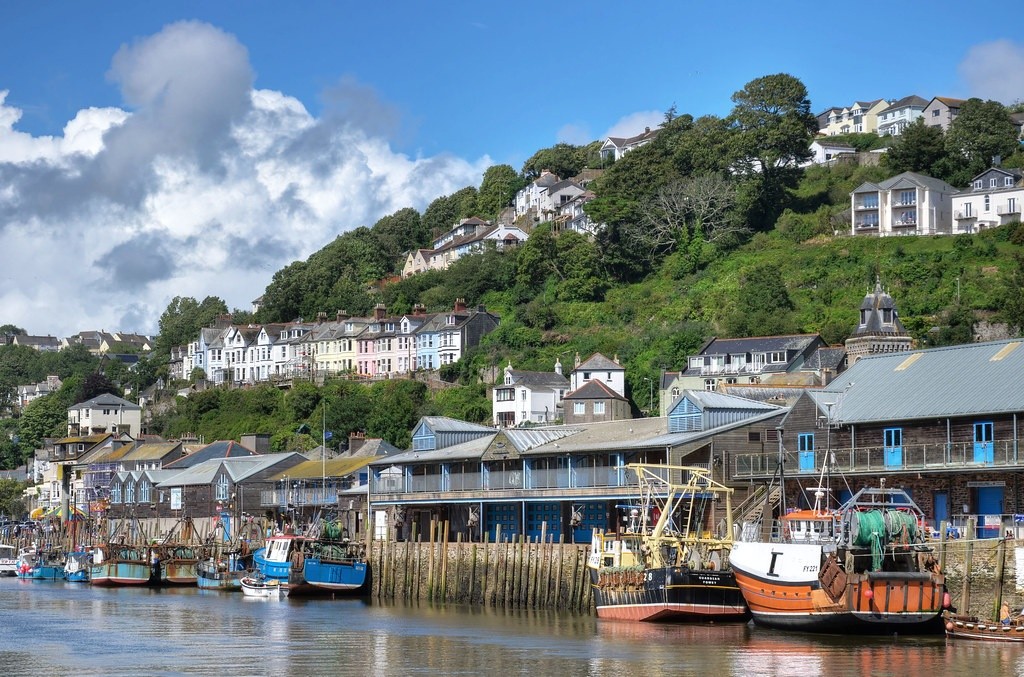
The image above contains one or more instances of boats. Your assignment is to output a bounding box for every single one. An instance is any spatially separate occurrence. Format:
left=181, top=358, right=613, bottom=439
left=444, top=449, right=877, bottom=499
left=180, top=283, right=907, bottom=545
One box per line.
left=728, top=399, right=946, bottom=632
left=942, top=610, right=1024, bottom=642
left=589, top=464, right=751, bottom=622
left=0, top=492, right=367, bottom=599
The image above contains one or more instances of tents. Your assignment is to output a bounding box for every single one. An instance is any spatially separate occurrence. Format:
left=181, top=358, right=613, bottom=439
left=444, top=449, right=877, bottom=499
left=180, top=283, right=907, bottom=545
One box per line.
left=38, top=504, right=88, bottom=519
